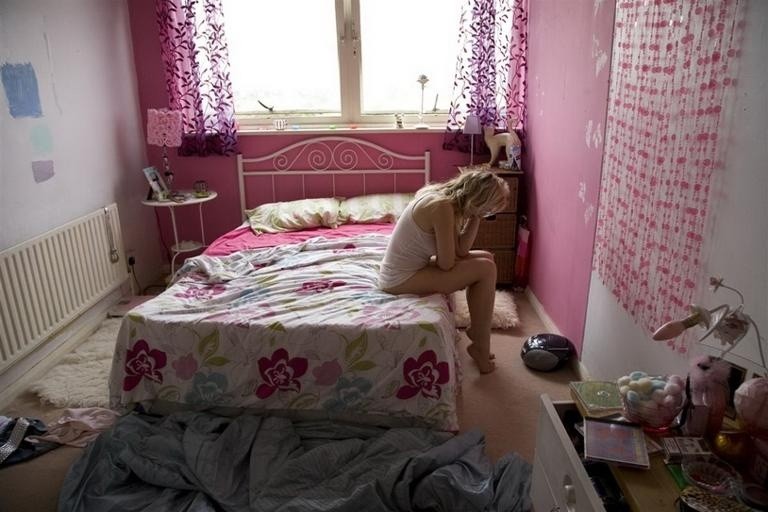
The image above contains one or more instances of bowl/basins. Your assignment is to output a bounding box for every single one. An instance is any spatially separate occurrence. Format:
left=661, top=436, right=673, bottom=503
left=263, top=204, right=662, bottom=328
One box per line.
left=619, top=375, right=687, bottom=430
left=679, top=453, right=745, bottom=498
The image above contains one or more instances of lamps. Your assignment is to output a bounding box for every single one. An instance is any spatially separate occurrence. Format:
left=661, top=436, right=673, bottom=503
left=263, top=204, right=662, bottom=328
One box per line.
left=649, top=275, right=768, bottom=370
left=463, top=113, right=481, bottom=163
left=146, top=107, right=184, bottom=204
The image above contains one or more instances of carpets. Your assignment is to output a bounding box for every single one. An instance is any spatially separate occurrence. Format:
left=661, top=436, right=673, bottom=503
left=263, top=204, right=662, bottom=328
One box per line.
left=24, top=310, right=121, bottom=409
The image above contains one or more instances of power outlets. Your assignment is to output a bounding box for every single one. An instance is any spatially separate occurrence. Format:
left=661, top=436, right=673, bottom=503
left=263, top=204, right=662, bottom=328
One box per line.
left=124, top=249, right=135, bottom=271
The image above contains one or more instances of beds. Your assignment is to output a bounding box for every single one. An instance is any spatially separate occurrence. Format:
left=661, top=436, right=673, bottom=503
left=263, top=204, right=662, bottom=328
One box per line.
left=106, top=137, right=462, bottom=416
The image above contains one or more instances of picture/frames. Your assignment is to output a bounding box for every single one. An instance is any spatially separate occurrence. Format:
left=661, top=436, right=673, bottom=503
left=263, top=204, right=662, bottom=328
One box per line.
left=143, top=166, right=167, bottom=200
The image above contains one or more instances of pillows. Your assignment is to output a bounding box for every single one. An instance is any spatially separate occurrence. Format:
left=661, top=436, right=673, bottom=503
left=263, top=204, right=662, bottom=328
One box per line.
left=240, top=193, right=348, bottom=235
left=342, top=190, right=420, bottom=223
left=456, top=290, right=523, bottom=333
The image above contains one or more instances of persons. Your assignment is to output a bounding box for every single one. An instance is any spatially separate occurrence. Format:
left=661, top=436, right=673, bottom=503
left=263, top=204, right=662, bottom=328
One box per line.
left=376, top=164, right=509, bottom=376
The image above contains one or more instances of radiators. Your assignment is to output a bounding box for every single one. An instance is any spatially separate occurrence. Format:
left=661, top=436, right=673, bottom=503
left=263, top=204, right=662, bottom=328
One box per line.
left=0, top=201, right=133, bottom=376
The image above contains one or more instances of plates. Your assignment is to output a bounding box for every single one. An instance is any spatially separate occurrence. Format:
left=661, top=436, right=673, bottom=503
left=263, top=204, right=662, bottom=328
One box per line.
left=193, top=193, right=210, bottom=198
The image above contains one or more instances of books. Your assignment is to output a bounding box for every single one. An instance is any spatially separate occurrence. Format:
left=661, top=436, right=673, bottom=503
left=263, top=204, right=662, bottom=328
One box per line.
left=568, top=377, right=627, bottom=418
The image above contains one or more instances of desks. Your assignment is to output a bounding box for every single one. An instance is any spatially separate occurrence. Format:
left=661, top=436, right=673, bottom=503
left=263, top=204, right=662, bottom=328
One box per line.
left=527, top=387, right=768, bottom=512
left=141, top=189, right=219, bottom=288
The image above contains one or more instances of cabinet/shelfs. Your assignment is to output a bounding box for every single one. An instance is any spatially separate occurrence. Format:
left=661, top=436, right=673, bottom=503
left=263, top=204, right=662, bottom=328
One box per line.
left=456, top=165, right=526, bottom=288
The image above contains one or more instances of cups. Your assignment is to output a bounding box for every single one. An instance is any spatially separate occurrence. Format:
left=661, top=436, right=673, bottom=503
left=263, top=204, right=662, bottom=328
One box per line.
left=195, top=181, right=207, bottom=192
